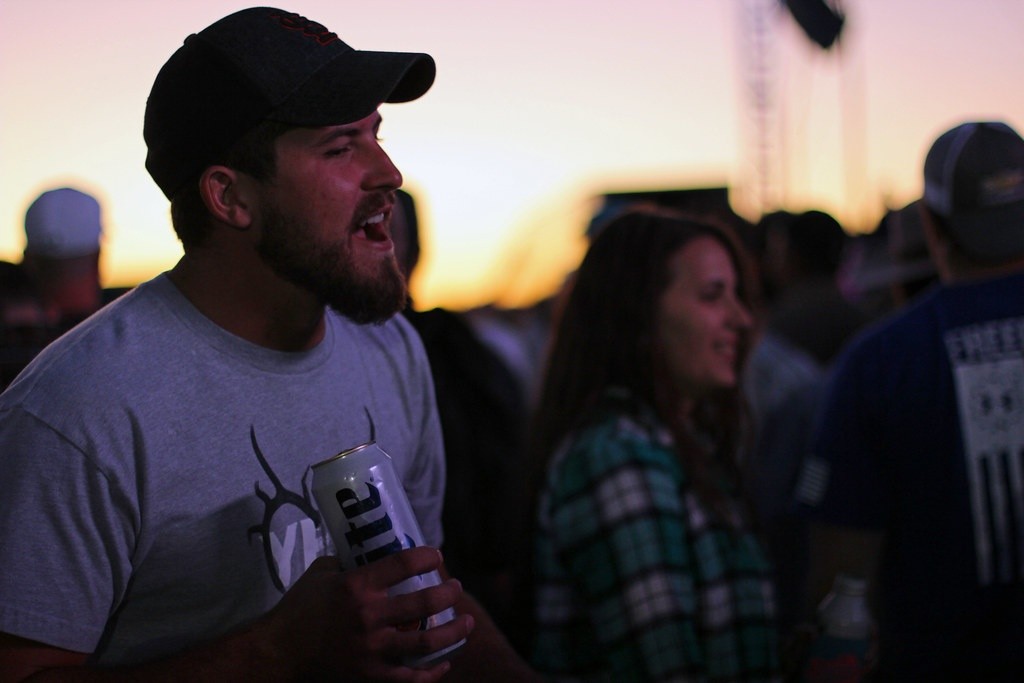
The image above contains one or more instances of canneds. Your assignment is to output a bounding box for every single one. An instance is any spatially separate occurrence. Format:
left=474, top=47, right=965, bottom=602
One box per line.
left=310, top=441, right=466, bottom=668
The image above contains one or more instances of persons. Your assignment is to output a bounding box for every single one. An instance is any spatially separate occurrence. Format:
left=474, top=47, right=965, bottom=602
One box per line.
left=0, top=5, right=543, bottom=683
left=0, top=121, right=1024, bottom=683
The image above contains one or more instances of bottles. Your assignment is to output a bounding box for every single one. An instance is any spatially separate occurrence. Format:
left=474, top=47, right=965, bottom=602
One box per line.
left=805, top=569, right=872, bottom=683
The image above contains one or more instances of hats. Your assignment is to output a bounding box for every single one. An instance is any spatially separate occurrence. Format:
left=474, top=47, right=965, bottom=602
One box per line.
left=25, top=188, right=100, bottom=257
left=144, top=7, right=436, bottom=204
left=923, top=123, right=1024, bottom=213
